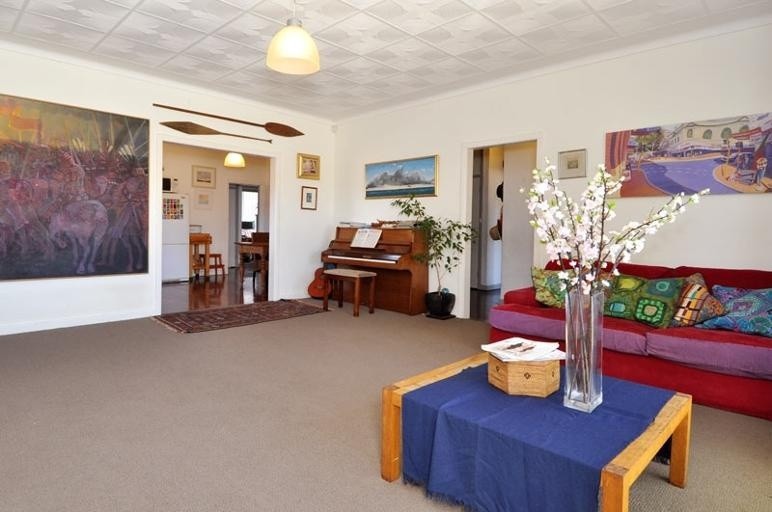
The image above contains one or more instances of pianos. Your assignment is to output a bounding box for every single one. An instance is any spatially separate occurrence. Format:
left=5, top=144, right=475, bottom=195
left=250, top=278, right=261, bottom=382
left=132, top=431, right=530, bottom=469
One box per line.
left=321, top=224, right=430, bottom=316
left=233, top=232, right=268, bottom=288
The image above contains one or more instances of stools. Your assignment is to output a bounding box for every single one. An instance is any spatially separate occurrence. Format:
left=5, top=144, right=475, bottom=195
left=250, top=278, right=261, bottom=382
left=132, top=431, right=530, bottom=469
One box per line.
left=323, top=268, right=377, bottom=317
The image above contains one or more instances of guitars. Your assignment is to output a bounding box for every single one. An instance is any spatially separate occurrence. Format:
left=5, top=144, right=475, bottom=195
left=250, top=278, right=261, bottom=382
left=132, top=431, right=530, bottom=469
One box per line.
left=308, top=263, right=332, bottom=298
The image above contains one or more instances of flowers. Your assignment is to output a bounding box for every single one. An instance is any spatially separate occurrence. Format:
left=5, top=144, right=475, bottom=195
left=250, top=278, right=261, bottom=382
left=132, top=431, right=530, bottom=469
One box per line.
left=518, top=155, right=710, bottom=402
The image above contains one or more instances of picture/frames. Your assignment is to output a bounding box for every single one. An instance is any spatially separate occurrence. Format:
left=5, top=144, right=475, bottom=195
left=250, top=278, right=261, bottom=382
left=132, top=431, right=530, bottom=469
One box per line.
left=557, top=148, right=588, bottom=180
left=297, top=152, right=321, bottom=180
left=362, top=154, right=440, bottom=200
left=191, top=164, right=217, bottom=189
left=301, top=186, right=318, bottom=210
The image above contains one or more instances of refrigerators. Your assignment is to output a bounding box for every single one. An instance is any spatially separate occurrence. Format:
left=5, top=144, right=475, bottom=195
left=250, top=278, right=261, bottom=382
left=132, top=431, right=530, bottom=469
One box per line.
left=162, top=193, right=191, bottom=284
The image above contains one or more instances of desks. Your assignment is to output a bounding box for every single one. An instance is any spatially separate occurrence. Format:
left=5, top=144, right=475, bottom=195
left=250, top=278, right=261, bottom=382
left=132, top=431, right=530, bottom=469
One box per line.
left=235, top=241, right=269, bottom=296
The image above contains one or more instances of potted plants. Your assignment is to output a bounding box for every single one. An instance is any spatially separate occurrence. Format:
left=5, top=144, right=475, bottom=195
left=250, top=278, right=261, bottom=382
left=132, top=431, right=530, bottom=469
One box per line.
left=390, top=191, right=481, bottom=320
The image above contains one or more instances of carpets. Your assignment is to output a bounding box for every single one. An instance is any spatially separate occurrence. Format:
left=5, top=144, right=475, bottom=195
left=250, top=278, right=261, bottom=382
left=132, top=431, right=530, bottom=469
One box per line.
left=151, top=299, right=332, bottom=336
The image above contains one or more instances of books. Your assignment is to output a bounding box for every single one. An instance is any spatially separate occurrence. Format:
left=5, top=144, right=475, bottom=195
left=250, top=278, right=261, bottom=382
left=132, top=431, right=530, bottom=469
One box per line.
left=350, top=228, right=383, bottom=248
left=480, top=336, right=574, bottom=363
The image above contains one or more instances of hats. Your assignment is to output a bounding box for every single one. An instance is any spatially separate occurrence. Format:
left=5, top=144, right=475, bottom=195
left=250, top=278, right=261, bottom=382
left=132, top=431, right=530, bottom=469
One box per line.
left=489, top=219, right=502, bottom=240
left=497, top=182, right=503, bottom=201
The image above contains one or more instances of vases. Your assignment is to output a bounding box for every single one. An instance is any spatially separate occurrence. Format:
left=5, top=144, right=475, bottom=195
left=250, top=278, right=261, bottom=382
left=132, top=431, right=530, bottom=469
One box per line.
left=563, top=292, right=605, bottom=414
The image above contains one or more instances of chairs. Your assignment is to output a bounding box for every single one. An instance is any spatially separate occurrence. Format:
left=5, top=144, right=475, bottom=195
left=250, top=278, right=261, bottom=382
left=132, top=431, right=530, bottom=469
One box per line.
left=251, top=231, right=269, bottom=294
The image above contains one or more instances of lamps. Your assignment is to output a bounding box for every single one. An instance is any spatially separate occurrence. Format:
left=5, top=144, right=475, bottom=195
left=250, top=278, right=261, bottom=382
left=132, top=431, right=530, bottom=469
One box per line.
left=224, top=152, right=246, bottom=167
left=266, top=1, right=320, bottom=76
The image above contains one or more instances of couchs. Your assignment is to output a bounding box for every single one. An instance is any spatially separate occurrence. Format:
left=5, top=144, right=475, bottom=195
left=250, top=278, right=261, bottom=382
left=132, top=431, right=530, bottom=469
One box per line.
left=488, top=257, right=771, bottom=423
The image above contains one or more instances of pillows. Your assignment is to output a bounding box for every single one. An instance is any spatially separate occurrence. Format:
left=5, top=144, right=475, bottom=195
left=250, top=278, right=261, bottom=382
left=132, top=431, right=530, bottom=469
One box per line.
left=531, top=265, right=771, bottom=340
